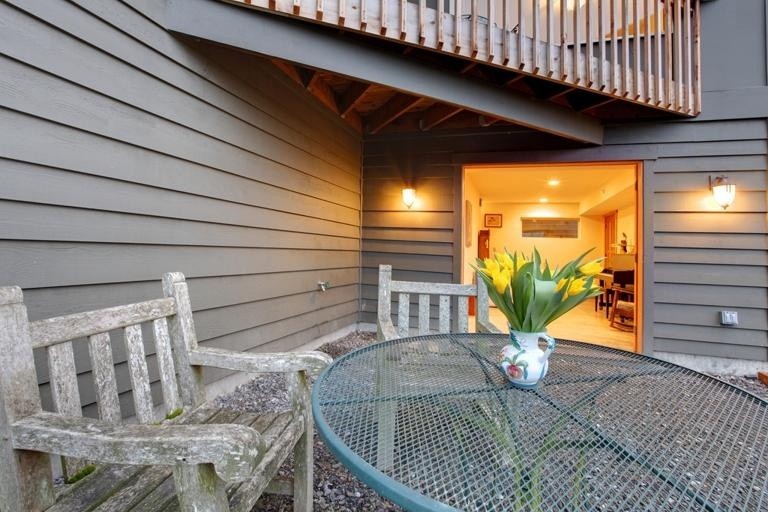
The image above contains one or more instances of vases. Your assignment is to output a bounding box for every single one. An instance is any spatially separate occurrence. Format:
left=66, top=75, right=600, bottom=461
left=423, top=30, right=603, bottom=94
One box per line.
left=499, top=330, right=556, bottom=391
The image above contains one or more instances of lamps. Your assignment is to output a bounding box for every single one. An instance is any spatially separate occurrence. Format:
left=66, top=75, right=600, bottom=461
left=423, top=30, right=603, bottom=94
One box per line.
left=401, top=181, right=416, bottom=208
left=712, top=176, right=736, bottom=209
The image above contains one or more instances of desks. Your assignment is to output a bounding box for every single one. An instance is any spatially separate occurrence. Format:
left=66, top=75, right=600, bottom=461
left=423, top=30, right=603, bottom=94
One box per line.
left=595, top=268, right=634, bottom=319
left=312, top=332, right=766, bottom=512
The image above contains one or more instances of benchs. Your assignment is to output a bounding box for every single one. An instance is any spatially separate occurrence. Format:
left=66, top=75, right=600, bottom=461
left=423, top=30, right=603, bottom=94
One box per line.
left=0, top=270, right=334, bottom=512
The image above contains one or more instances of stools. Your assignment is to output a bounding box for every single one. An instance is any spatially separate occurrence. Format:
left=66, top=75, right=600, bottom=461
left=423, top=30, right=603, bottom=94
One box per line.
left=610, top=287, right=634, bottom=329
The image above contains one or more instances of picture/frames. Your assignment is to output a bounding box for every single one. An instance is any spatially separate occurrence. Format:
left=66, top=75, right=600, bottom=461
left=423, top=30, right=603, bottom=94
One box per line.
left=484, top=213, right=502, bottom=227
left=466, top=200, right=472, bottom=246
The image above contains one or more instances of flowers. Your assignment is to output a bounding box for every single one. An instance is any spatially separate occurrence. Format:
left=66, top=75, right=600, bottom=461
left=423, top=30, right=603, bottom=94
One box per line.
left=469, top=247, right=606, bottom=331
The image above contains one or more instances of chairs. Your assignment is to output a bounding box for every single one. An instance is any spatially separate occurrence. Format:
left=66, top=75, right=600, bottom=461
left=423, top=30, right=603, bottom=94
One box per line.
left=378, top=264, right=520, bottom=471
